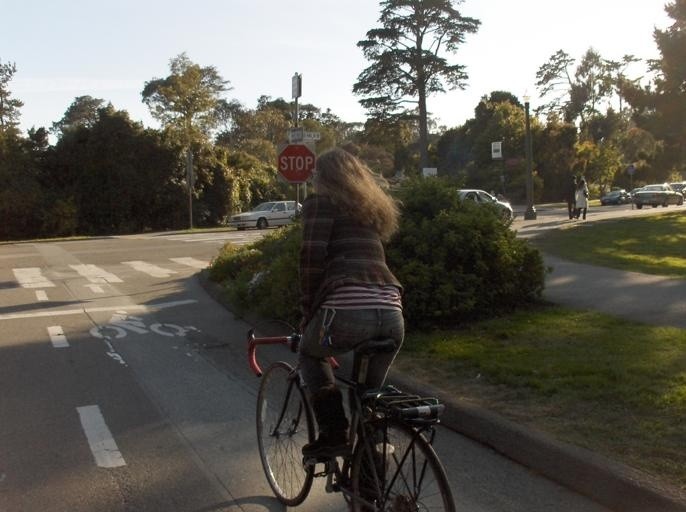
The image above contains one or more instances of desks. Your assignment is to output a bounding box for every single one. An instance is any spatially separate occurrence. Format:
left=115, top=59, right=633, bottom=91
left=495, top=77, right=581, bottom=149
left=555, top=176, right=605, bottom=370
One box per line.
left=522, top=89, right=537, bottom=220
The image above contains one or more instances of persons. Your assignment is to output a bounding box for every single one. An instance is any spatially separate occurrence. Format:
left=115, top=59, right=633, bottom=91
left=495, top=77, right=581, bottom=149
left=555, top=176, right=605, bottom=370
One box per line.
left=563, top=176, right=577, bottom=219
left=575, top=180, right=589, bottom=220
left=295, top=148, right=405, bottom=512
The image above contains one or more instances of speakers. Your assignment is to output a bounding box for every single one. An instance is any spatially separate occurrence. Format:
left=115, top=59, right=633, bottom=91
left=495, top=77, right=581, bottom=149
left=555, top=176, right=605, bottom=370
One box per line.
left=301, top=385, right=351, bottom=458
left=356, top=418, right=393, bottom=511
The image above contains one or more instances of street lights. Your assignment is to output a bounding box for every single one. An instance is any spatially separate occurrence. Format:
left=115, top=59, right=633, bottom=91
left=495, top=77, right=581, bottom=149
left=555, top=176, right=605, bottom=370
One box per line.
left=584, top=186, right=590, bottom=197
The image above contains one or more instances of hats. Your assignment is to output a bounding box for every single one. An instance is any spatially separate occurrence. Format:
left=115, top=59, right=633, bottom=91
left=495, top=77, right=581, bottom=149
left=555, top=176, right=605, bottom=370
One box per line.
left=456, top=189, right=514, bottom=223
left=227, top=200, right=302, bottom=231
left=602, top=182, right=686, bottom=209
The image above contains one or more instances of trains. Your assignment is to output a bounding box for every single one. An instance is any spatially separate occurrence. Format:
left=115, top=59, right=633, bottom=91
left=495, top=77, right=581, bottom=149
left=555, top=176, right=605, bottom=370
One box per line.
left=277, top=143, right=316, bottom=183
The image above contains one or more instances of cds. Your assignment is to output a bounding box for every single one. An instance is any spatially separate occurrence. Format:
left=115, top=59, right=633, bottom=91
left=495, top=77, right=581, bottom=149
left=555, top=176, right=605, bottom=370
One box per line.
left=318, top=308, right=336, bottom=349
